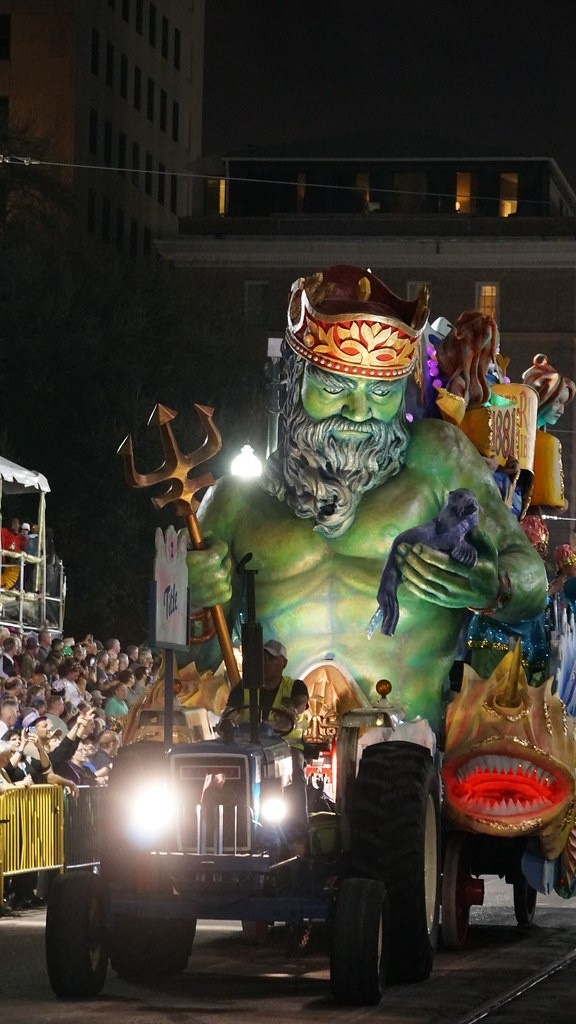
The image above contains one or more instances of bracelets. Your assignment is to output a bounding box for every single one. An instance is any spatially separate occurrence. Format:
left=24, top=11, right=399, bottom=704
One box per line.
left=293, top=708, right=298, bottom=714
left=17, top=750, right=23, bottom=754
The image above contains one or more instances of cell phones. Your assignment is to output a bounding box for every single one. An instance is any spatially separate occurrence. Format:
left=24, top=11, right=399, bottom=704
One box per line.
left=28, top=727, right=36, bottom=734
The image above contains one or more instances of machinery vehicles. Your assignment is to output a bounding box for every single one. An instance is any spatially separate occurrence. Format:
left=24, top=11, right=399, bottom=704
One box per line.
left=45, top=569, right=445, bottom=1006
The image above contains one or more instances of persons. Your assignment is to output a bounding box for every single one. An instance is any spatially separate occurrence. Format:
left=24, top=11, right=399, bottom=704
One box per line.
left=0, top=514, right=163, bottom=916
left=493, top=354, right=576, bottom=522
left=185, top=296, right=549, bottom=752
left=425, top=306, right=521, bottom=476
left=222, top=639, right=310, bottom=859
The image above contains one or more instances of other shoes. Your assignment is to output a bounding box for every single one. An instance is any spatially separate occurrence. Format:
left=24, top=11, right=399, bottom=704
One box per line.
left=17, top=892, right=43, bottom=907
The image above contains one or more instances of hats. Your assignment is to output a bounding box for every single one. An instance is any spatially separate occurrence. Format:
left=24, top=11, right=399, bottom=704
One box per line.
left=25, top=637, right=40, bottom=651
left=91, top=690, right=107, bottom=700
left=21, top=713, right=47, bottom=730
left=264, top=639, right=288, bottom=668
